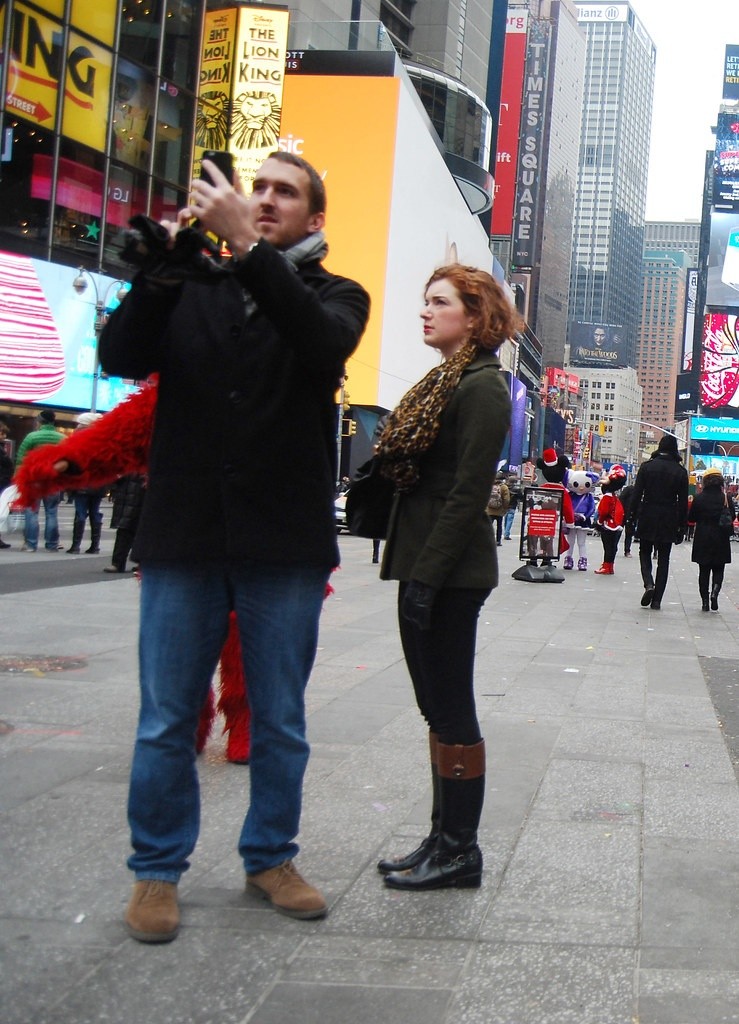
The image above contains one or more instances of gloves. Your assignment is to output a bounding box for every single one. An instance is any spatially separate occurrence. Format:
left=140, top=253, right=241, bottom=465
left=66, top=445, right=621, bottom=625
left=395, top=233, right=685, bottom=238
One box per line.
left=674, top=533, right=683, bottom=545
left=626, top=521, right=636, bottom=536
left=400, top=581, right=438, bottom=633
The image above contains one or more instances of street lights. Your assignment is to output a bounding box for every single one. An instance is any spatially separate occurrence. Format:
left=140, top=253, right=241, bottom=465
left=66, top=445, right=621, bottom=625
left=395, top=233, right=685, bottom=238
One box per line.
left=72, top=261, right=131, bottom=421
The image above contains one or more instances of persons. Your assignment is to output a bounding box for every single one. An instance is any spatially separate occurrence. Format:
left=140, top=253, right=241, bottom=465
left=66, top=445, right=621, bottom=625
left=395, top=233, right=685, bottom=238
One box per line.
left=98, top=150, right=372, bottom=942
left=377, top=266, right=524, bottom=890
left=0, top=409, right=144, bottom=574
left=584, top=324, right=615, bottom=361
left=618, top=483, right=739, bottom=559
left=488, top=471, right=522, bottom=547
left=687, top=469, right=736, bottom=611
left=630, top=436, right=689, bottom=610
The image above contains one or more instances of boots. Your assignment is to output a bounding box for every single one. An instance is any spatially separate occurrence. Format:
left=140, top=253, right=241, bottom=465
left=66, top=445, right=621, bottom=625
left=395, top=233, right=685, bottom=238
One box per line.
left=378, top=736, right=486, bottom=892
left=700, top=583, right=721, bottom=612
left=595, top=562, right=614, bottom=575
left=66, top=521, right=102, bottom=554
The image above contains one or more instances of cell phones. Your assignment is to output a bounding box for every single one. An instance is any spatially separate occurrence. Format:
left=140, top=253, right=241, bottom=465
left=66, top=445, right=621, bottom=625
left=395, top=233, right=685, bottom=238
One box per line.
left=199, top=150, right=233, bottom=187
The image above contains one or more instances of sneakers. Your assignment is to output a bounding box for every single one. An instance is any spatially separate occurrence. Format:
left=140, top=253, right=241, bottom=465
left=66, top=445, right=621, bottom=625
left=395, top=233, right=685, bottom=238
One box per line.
left=245, top=862, right=328, bottom=919
left=125, top=880, right=177, bottom=943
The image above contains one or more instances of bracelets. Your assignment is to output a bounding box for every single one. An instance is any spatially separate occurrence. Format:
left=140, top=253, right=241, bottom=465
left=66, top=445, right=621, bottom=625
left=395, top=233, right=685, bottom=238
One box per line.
left=248, top=242, right=259, bottom=253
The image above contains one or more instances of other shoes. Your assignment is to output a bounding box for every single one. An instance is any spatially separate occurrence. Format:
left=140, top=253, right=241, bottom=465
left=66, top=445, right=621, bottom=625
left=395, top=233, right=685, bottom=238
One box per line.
left=578, top=556, right=587, bottom=570
left=641, top=587, right=654, bottom=606
left=564, top=556, right=573, bottom=569
left=102, top=565, right=140, bottom=574
left=497, top=542, right=502, bottom=546
left=0, top=541, right=61, bottom=552
left=625, top=553, right=632, bottom=558
left=650, top=602, right=660, bottom=610
left=505, top=537, right=511, bottom=540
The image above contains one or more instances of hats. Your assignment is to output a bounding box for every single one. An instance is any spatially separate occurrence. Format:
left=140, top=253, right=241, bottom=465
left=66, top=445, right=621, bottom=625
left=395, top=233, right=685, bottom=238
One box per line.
left=73, top=412, right=104, bottom=425
left=37, top=410, right=56, bottom=424
left=704, top=468, right=724, bottom=480
left=496, top=473, right=503, bottom=480
left=658, top=434, right=678, bottom=454
left=509, top=476, right=516, bottom=483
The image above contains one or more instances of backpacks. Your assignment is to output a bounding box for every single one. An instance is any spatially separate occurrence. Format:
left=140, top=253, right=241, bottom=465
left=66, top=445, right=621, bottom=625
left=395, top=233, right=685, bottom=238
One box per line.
left=486, top=483, right=504, bottom=509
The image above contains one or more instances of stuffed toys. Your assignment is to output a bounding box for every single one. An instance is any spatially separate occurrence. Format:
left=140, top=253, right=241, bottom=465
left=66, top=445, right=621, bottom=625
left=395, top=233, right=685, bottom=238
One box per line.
left=16, top=369, right=340, bottom=764
left=537, top=449, right=627, bottom=574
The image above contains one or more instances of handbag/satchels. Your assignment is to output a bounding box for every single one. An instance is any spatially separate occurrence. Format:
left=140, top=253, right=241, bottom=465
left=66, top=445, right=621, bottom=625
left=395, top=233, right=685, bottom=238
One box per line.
left=719, top=492, right=736, bottom=536
left=347, top=444, right=393, bottom=541
